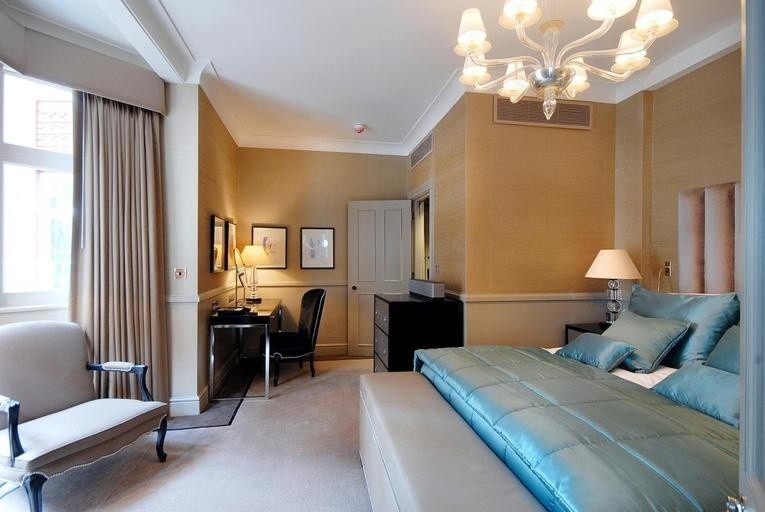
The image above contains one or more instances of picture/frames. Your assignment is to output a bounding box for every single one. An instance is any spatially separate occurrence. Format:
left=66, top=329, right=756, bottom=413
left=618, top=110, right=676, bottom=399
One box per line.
left=300, top=226, right=335, bottom=268
left=211, top=214, right=226, bottom=272
left=225, top=221, right=237, bottom=271
left=251, top=225, right=287, bottom=269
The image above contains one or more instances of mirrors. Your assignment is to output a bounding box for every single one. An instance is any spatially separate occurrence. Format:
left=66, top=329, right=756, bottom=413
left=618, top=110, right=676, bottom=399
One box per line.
left=232, top=247, right=247, bottom=307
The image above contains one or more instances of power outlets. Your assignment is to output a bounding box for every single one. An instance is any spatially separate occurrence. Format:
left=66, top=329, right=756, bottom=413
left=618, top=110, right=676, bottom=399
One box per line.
left=663, top=261, right=673, bottom=278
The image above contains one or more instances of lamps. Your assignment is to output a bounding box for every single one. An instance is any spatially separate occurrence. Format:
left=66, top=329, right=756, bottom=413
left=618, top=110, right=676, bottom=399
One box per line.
left=585, top=247, right=643, bottom=325
left=242, top=244, right=270, bottom=303
left=455, top=0, right=678, bottom=120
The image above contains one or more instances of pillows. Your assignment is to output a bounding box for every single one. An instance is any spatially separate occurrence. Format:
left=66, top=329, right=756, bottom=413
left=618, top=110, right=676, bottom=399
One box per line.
left=554, top=331, right=637, bottom=373
left=651, top=361, right=740, bottom=430
left=599, top=307, right=691, bottom=374
left=630, top=284, right=740, bottom=367
left=708, top=323, right=738, bottom=374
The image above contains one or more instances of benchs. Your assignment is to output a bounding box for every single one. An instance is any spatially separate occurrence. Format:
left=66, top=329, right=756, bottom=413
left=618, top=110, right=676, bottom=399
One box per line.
left=1, top=320, right=170, bottom=512
left=356, top=371, right=548, bottom=512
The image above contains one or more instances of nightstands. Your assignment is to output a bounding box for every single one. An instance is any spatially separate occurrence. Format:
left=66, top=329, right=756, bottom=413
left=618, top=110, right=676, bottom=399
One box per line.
left=564, top=322, right=612, bottom=347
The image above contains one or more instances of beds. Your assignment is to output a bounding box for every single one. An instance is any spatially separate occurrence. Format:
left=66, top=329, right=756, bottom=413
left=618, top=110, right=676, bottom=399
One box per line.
left=412, top=288, right=739, bottom=512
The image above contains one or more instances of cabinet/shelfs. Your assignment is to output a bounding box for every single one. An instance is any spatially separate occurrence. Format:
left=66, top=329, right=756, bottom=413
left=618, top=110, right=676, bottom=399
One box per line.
left=371, top=292, right=464, bottom=371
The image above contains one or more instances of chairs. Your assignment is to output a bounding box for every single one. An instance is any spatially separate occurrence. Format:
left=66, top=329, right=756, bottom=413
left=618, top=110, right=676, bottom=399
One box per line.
left=258, top=287, right=326, bottom=387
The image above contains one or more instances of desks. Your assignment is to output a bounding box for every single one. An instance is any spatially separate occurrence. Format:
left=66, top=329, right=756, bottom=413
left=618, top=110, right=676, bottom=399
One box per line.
left=208, top=298, right=285, bottom=401
